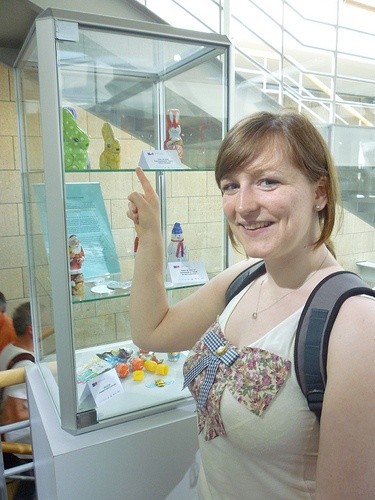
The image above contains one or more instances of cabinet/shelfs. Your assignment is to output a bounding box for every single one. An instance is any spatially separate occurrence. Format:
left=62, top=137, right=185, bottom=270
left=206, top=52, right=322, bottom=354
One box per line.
left=13, top=6, right=233, bottom=435
left=22, top=365, right=203, bottom=500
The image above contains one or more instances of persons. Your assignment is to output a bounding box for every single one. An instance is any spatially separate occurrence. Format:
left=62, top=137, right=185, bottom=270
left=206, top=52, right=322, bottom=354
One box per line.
left=0, top=302, right=36, bottom=500
left=68, top=235, right=85, bottom=296
left=126, top=110, right=375, bottom=499
left=0, top=292, right=17, bottom=353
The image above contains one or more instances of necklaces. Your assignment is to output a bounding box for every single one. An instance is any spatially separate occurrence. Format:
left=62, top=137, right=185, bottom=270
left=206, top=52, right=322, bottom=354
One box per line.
left=252, top=249, right=328, bottom=319
